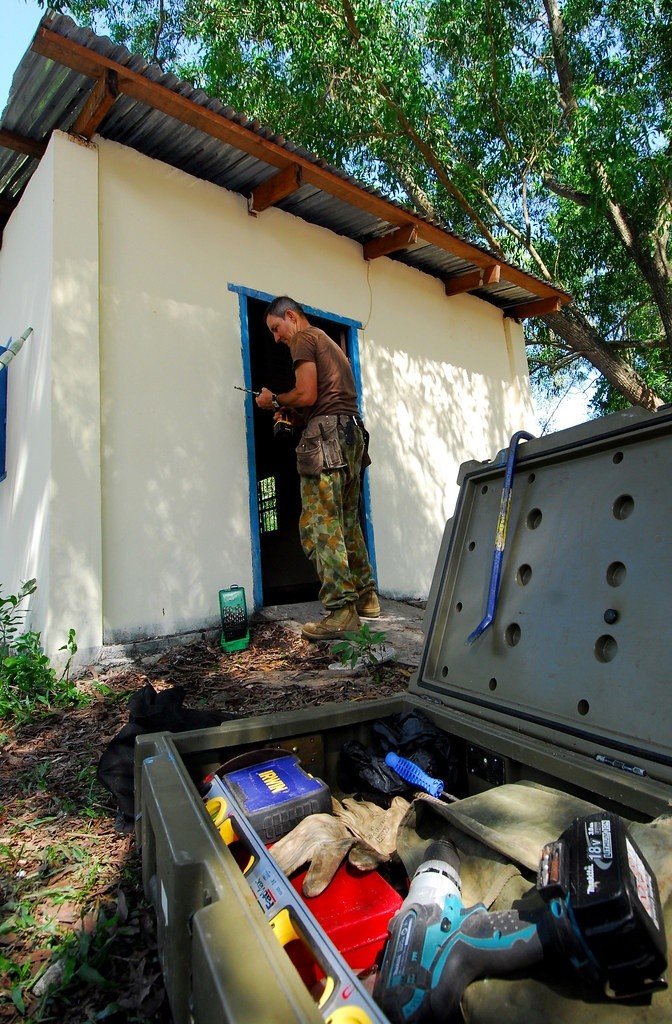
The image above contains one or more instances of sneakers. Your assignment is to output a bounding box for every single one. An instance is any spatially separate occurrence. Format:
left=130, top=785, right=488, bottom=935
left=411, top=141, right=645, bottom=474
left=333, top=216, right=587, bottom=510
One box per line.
left=301, top=602, right=362, bottom=640
left=356, top=591, right=381, bottom=618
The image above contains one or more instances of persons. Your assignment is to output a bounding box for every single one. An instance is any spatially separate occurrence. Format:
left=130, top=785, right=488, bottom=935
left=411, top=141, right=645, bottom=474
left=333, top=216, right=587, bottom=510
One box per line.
left=256, top=297, right=380, bottom=640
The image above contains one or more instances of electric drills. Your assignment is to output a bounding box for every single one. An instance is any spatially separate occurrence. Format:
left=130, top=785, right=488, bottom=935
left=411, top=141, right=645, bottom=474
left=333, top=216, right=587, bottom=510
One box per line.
left=233, top=385, right=294, bottom=439
left=371, top=811, right=668, bottom=1024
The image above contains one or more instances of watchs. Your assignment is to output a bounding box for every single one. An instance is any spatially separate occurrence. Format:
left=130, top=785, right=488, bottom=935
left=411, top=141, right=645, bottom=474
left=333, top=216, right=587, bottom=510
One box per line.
left=272, top=394, right=281, bottom=410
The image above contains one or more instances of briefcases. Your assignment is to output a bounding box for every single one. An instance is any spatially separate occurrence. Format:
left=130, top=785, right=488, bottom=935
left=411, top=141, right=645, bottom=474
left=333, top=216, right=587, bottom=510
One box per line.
left=133, top=404, right=672, bottom=1024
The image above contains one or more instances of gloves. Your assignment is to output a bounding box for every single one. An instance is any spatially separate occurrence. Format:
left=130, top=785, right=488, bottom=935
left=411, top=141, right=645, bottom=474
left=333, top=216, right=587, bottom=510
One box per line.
left=269, top=795, right=412, bottom=899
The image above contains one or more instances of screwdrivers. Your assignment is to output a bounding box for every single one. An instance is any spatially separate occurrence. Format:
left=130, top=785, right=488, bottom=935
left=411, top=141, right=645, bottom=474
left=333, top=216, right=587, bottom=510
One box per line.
left=385, top=751, right=460, bottom=802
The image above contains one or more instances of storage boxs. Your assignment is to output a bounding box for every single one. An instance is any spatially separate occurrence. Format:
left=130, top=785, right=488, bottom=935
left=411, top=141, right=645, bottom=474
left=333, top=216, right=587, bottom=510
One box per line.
left=221, top=751, right=334, bottom=845
left=220, top=585, right=249, bottom=651
left=265, top=842, right=404, bottom=985
